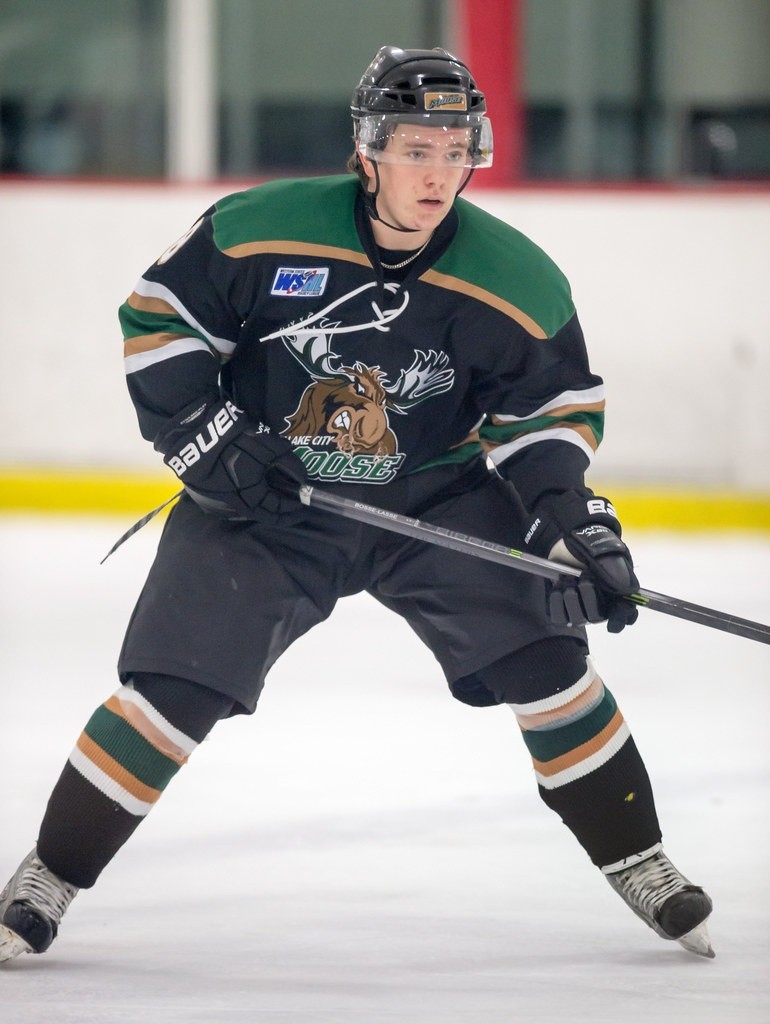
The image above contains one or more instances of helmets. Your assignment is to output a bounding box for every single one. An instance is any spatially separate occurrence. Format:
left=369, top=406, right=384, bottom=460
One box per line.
left=350, top=45, right=488, bottom=155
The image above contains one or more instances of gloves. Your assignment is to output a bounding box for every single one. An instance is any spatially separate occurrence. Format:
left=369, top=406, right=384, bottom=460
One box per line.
left=517, top=486, right=640, bottom=634
left=154, top=388, right=315, bottom=529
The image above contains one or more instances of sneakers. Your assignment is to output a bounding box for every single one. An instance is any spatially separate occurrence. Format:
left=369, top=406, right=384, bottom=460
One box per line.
left=1, top=844, right=79, bottom=966
left=605, top=848, right=718, bottom=962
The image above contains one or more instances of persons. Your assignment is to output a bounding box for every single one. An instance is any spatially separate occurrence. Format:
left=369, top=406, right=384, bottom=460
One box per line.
left=0, top=42, right=719, bottom=975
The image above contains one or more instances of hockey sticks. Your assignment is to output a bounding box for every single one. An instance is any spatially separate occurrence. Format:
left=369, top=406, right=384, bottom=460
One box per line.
left=299, top=486, right=770, bottom=647
